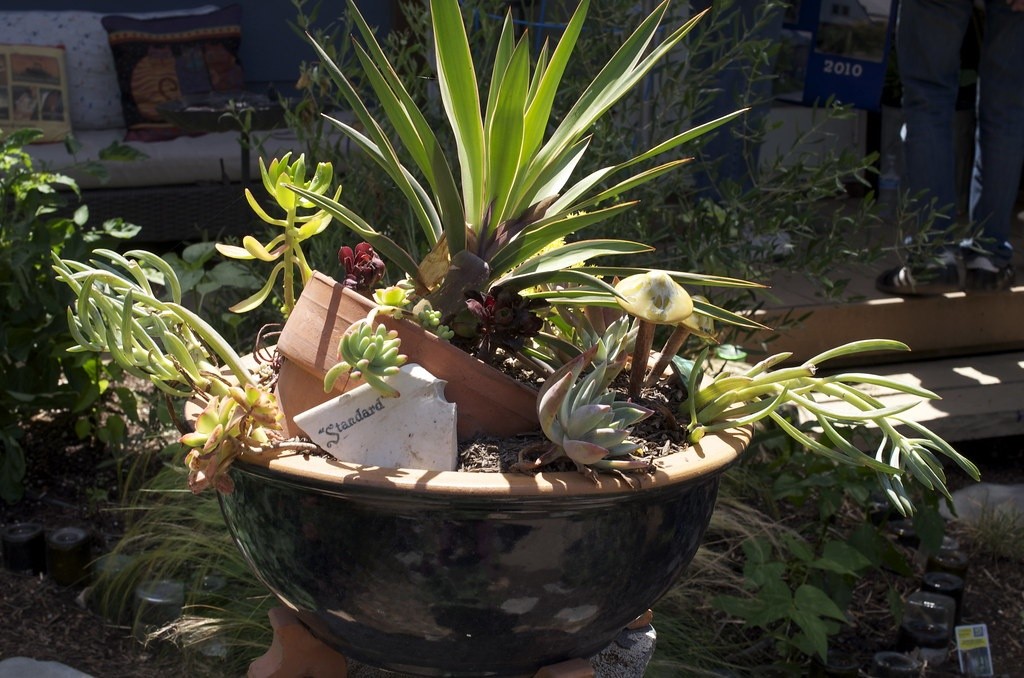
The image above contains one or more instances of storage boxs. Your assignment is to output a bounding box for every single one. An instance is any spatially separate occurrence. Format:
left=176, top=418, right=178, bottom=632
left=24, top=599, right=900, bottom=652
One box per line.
left=757, top=106, right=868, bottom=182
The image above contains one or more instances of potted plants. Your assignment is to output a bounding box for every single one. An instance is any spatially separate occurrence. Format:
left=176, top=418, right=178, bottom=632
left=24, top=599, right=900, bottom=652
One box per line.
left=49, top=149, right=985, bottom=678
left=269, top=0, right=753, bottom=442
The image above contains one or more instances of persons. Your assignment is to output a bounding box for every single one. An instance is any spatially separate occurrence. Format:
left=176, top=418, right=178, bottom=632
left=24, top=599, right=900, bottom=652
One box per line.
left=877, top=0, right=1024, bottom=295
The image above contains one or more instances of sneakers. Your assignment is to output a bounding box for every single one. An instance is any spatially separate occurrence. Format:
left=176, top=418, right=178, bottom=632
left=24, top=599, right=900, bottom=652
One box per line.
left=874, top=258, right=960, bottom=295
left=962, top=265, right=1018, bottom=297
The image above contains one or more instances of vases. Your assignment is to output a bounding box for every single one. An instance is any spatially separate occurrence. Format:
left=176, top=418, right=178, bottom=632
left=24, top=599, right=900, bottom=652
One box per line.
left=2, top=524, right=258, bottom=678
left=729, top=471, right=971, bottom=678
